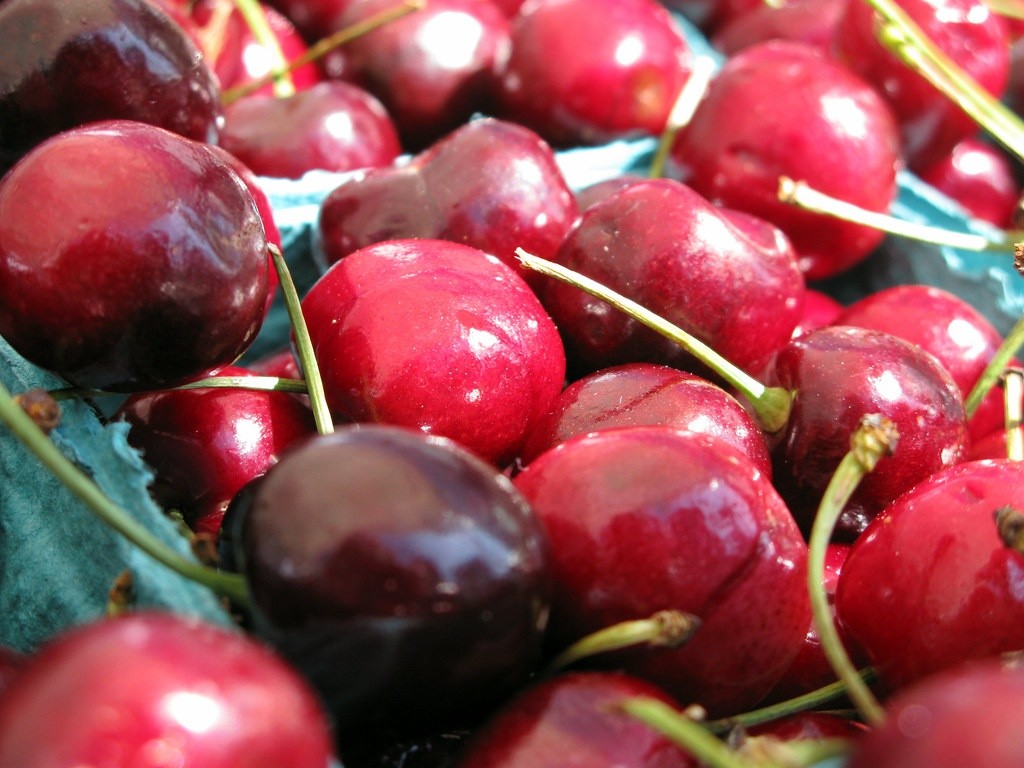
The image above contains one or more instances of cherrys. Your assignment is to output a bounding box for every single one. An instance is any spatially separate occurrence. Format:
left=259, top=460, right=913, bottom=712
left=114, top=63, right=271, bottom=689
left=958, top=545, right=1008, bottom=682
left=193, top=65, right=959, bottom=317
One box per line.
left=0, top=1, right=1024, bottom=768
